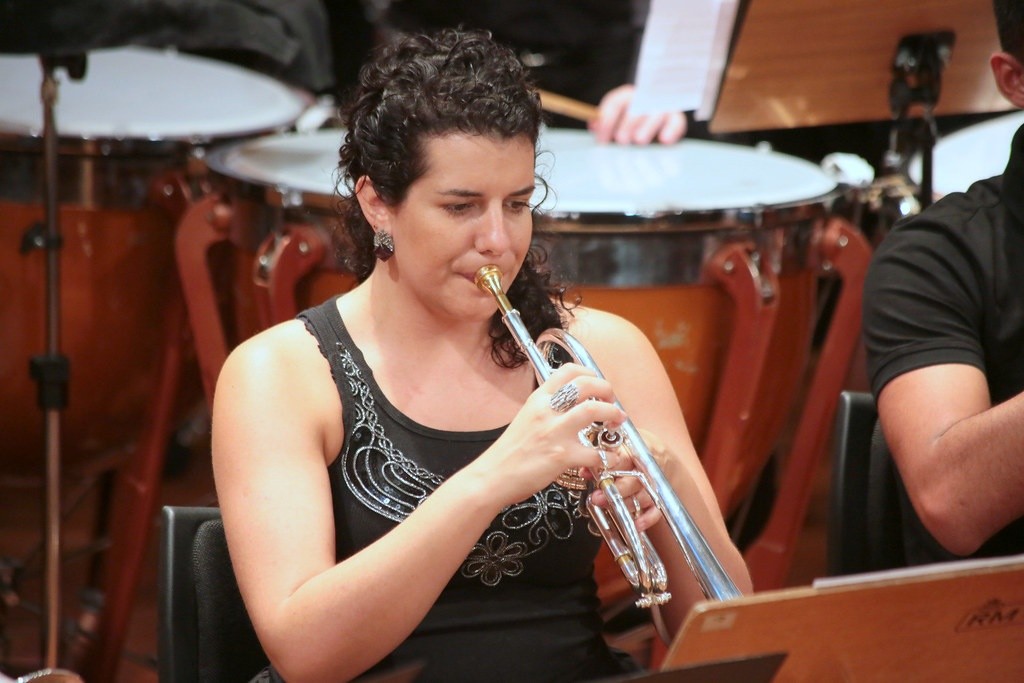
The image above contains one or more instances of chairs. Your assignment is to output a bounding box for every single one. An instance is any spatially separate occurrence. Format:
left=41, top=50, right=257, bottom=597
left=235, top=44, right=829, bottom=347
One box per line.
left=157, top=506, right=354, bottom=683
left=825, top=390, right=968, bottom=576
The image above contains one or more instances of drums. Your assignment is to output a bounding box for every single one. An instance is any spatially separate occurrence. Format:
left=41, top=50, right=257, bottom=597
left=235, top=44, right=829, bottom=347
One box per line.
left=0, top=43, right=324, bottom=558
left=189, top=128, right=846, bottom=535
left=895, top=107, right=1024, bottom=224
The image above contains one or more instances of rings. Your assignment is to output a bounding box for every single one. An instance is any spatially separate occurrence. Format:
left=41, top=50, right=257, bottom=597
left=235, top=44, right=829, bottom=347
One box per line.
left=549, top=382, right=581, bottom=413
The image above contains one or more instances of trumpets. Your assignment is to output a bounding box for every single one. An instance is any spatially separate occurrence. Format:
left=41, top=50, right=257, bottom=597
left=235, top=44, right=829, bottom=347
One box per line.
left=473, top=263, right=744, bottom=613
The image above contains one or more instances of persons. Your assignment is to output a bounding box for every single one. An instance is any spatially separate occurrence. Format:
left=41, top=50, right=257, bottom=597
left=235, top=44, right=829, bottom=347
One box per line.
left=850, top=0, right=1023, bottom=572
left=210, top=23, right=755, bottom=682
left=344, top=0, right=751, bottom=148
